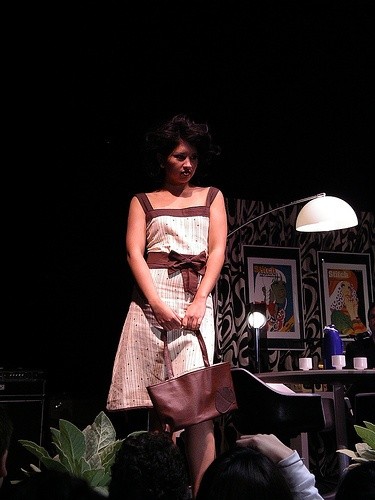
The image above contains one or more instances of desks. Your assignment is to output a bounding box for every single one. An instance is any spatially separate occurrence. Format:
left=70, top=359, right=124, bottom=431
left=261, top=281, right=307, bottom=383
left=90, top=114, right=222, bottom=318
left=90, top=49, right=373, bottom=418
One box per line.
left=254, top=369, right=375, bottom=500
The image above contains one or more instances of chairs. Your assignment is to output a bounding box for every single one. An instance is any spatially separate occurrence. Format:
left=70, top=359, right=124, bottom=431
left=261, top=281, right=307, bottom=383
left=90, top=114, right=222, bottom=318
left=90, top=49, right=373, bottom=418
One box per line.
left=229, top=366, right=334, bottom=448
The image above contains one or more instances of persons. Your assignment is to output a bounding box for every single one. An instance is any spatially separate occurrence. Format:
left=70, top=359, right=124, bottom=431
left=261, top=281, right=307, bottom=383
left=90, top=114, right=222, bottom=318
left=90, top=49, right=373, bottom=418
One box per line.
left=195, top=434, right=326, bottom=500
left=346, top=302, right=375, bottom=369
left=109, top=428, right=190, bottom=500
left=0, top=409, right=96, bottom=500
left=106, top=110, right=227, bottom=500
left=334, top=461, right=375, bottom=500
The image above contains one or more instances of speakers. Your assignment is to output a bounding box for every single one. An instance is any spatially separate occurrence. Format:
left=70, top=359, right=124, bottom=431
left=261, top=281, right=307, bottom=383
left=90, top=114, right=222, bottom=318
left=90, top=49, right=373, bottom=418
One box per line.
left=1, top=401, right=45, bottom=483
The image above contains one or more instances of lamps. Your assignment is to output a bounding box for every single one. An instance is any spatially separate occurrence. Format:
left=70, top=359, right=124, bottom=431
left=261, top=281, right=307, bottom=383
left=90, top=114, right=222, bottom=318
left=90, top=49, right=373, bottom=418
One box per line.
left=227, top=192, right=359, bottom=238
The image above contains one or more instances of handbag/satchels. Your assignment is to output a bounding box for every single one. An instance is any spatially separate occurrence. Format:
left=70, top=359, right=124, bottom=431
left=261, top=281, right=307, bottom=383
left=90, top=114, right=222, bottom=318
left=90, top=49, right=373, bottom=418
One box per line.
left=146, top=322, right=239, bottom=432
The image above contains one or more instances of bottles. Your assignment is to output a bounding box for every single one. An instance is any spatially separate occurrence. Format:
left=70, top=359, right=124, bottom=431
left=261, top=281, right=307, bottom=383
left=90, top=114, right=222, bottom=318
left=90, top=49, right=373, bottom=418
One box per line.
left=324, top=324, right=342, bottom=369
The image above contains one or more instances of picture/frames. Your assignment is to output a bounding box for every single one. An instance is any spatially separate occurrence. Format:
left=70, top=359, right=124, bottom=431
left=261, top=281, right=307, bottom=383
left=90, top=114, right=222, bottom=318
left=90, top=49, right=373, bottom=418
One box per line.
left=317, top=250, right=374, bottom=341
left=241, top=244, right=307, bottom=351
